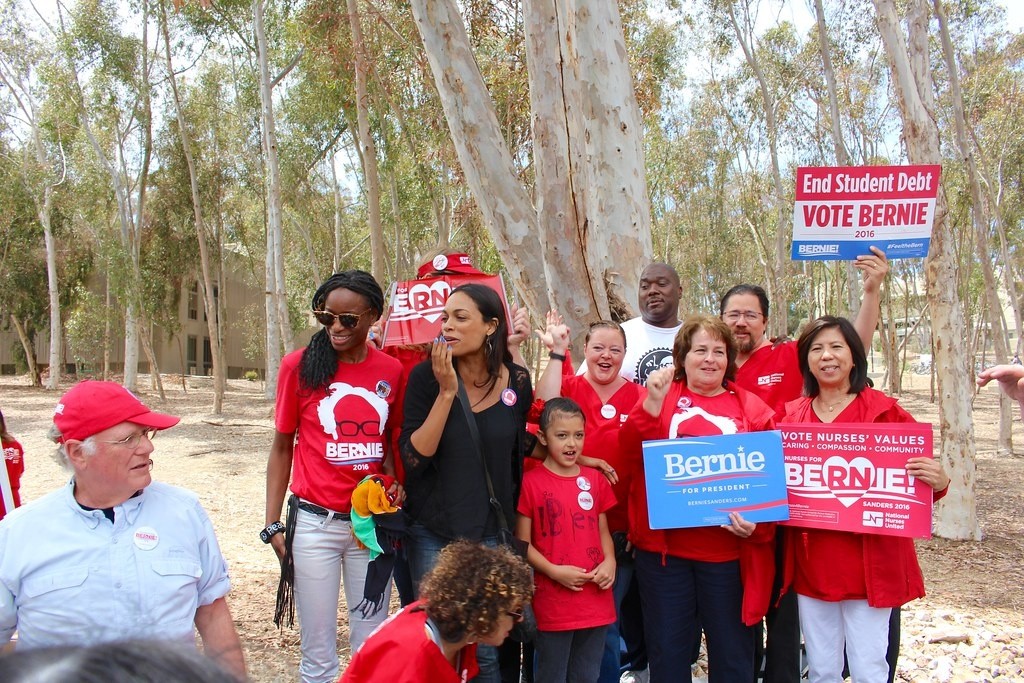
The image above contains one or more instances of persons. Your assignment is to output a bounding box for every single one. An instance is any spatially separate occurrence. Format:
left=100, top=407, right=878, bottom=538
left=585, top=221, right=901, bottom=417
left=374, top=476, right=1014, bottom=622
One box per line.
left=264, top=269, right=409, bottom=683
left=526, top=320, right=647, bottom=683
left=337, top=539, right=533, bottom=683
left=618, top=314, right=777, bottom=683
left=0, top=380, right=247, bottom=683
left=516, top=397, right=617, bottom=683
left=718, top=246, right=888, bottom=683
left=366, top=247, right=531, bottom=610
left=780, top=315, right=951, bottom=683
left=397, top=282, right=618, bottom=683
left=537, top=263, right=683, bottom=388
left=976, top=364, right=1024, bottom=421
left=0, top=410, right=25, bottom=521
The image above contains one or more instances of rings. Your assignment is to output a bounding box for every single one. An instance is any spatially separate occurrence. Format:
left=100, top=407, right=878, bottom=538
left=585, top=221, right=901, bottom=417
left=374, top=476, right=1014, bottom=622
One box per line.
left=873, top=264, right=876, bottom=269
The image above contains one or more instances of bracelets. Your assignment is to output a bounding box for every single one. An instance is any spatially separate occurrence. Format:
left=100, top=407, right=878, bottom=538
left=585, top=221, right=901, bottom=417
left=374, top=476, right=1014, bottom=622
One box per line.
left=259, top=521, right=286, bottom=544
left=548, top=350, right=567, bottom=362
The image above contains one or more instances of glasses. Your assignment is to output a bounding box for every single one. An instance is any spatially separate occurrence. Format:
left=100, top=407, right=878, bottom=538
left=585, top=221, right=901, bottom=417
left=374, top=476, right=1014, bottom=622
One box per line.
left=335, top=420, right=380, bottom=436
left=92, top=426, right=158, bottom=447
left=315, top=304, right=378, bottom=328
left=504, top=608, right=523, bottom=621
left=722, top=310, right=764, bottom=321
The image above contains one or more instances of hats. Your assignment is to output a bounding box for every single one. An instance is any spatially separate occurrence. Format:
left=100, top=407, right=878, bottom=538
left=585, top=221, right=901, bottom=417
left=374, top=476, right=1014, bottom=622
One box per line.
left=53, top=380, right=180, bottom=443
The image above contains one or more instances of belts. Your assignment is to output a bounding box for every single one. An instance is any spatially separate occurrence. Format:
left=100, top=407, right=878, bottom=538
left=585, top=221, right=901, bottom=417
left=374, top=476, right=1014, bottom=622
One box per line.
left=299, top=503, right=350, bottom=521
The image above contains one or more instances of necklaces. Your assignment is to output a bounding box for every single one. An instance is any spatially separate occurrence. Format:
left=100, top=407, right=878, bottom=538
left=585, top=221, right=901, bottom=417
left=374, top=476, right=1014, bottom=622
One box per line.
left=818, top=394, right=850, bottom=412
